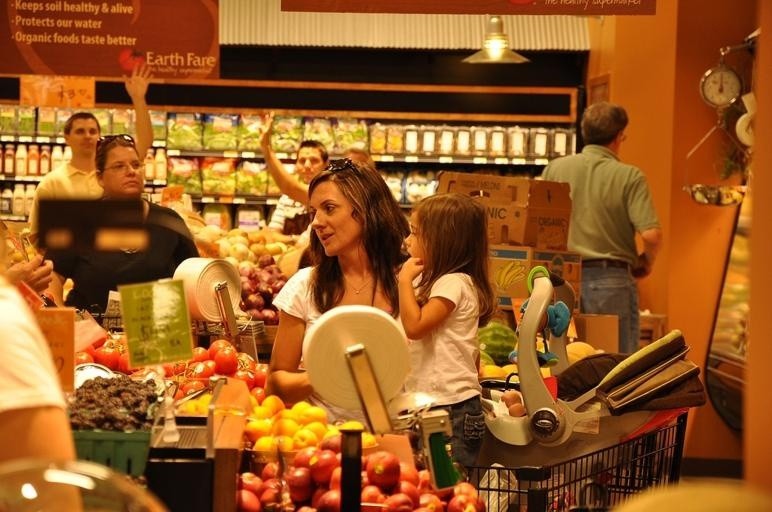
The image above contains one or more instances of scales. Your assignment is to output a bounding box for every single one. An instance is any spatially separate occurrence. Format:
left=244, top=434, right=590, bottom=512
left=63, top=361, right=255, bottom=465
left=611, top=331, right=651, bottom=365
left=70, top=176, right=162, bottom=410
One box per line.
left=684, top=45, right=750, bottom=206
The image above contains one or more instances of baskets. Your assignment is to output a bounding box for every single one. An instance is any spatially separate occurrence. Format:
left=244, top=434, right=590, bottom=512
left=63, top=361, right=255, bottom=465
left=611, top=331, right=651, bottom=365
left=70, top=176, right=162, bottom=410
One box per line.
left=73, top=429, right=151, bottom=479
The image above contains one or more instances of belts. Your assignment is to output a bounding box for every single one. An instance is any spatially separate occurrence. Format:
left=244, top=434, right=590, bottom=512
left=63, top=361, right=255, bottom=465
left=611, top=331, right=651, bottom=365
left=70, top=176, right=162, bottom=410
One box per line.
left=581, top=258, right=627, bottom=268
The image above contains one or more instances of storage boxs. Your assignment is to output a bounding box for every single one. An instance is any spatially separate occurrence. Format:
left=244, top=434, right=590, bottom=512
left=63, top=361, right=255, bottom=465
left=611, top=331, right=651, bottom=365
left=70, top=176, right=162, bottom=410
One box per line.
left=437, top=172, right=572, bottom=251
left=484, top=255, right=583, bottom=312
left=517, top=315, right=620, bottom=357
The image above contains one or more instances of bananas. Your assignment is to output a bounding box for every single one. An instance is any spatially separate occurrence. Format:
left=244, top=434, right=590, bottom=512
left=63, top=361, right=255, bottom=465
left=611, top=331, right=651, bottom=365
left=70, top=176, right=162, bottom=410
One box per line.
left=494, top=261, right=525, bottom=290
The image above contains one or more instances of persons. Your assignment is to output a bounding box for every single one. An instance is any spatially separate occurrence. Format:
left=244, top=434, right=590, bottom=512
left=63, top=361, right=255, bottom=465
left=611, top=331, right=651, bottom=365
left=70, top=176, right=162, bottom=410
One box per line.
left=263, top=157, right=430, bottom=433
left=396, top=190, right=496, bottom=484
left=0, top=218, right=66, bottom=310
left=63, top=133, right=203, bottom=315
left=255, top=109, right=380, bottom=209
left=267, top=138, right=330, bottom=232
left=0, top=273, right=90, bottom=511
left=26, top=62, right=156, bottom=240
left=538, top=101, right=664, bottom=355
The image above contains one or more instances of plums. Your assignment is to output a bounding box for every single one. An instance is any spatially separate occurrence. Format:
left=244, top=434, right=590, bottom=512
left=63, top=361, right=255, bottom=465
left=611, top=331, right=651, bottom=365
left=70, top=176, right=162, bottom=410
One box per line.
left=238, top=254, right=288, bottom=326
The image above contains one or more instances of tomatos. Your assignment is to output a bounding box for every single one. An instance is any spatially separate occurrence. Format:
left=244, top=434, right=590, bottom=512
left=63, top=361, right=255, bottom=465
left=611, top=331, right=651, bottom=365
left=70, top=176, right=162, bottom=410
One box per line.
left=74, top=333, right=267, bottom=405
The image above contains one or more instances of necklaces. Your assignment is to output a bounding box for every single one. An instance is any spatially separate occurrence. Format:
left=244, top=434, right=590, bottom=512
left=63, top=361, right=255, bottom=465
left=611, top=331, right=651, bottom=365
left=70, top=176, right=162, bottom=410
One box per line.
left=344, top=276, right=373, bottom=295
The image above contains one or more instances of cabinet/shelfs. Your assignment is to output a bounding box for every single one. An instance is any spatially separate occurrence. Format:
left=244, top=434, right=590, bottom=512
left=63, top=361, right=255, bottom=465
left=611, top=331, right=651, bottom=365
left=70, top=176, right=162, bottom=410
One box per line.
left=0, top=101, right=166, bottom=255
left=369, top=122, right=579, bottom=241
left=164, top=111, right=367, bottom=235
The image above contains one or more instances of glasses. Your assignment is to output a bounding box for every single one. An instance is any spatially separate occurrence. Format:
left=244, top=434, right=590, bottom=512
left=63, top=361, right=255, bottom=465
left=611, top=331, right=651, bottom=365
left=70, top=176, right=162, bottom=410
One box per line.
left=326, top=157, right=361, bottom=177
left=621, top=133, right=627, bottom=142
left=95, top=135, right=135, bottom=150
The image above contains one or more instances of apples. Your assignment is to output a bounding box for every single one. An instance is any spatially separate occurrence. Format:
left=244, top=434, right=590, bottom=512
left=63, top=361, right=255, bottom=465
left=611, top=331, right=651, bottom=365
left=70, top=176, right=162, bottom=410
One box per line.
left=238, top=394, right=485, bottom=512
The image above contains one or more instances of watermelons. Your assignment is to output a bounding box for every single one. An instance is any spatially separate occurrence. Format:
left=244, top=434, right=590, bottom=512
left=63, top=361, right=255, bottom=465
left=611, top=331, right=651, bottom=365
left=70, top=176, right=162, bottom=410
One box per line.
left=477, top=322, right=518, bottom=365
left=479, top=350, right=497, bottom=365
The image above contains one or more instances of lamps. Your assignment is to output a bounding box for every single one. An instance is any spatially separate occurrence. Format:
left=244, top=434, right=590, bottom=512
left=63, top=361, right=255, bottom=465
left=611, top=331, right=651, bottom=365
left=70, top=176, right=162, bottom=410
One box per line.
left=458, top=13, right=531, bottom=63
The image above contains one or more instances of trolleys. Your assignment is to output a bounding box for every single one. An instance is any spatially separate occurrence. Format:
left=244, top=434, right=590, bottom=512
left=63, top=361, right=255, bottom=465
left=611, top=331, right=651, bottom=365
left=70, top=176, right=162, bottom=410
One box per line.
left=451, top=397, right=688, bottom=511
